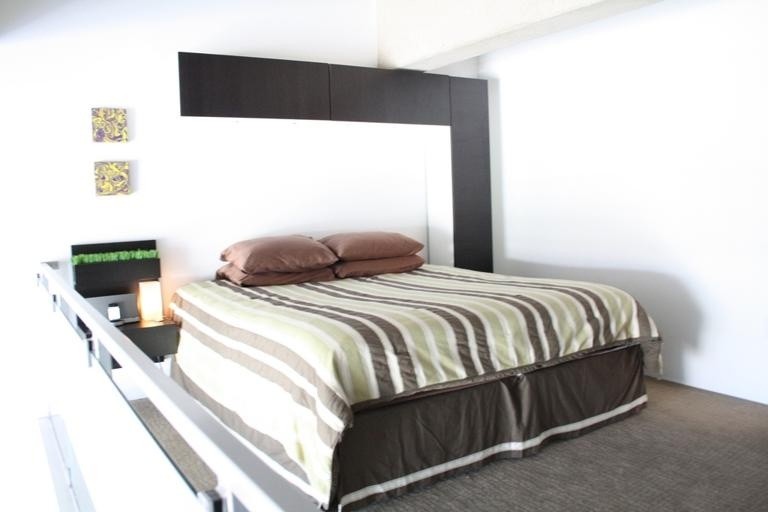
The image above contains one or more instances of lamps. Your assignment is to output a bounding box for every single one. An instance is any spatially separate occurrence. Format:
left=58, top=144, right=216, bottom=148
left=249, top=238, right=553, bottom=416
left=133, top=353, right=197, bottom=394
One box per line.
left=212, top=264, right=335, bottom=287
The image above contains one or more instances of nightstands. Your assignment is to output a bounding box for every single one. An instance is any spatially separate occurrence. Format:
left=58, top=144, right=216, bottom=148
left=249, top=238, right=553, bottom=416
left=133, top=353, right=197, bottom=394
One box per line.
left=79, top=315, right=181, bottom=401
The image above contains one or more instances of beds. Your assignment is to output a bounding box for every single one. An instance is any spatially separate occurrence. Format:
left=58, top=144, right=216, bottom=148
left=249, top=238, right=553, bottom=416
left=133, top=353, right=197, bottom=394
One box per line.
left=163, top=257, right=665, bottom=512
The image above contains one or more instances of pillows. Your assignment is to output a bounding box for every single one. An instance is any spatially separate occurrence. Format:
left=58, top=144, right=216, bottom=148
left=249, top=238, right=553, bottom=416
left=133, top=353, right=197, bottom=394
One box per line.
left=219, top=235, right=340, bottom=273
left=318, top=231, right=423, bottom=262
left=328, top=255, right=425, bottom=279
